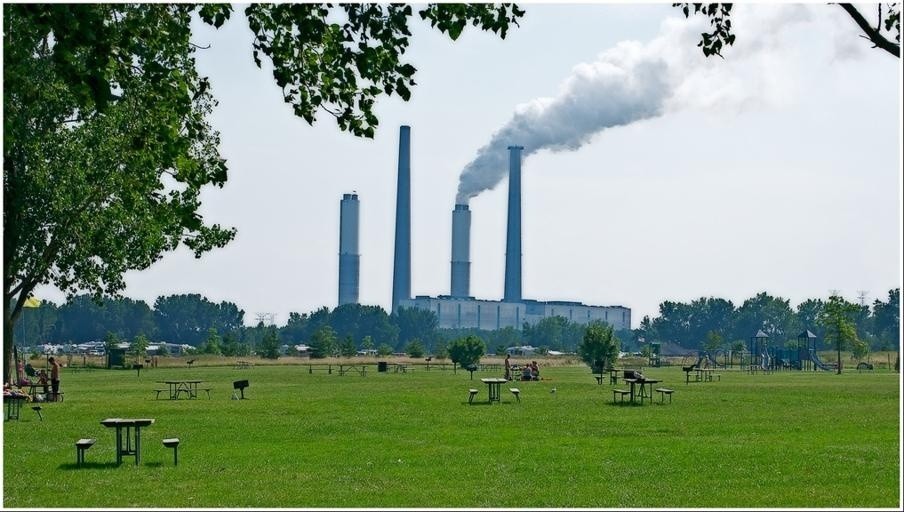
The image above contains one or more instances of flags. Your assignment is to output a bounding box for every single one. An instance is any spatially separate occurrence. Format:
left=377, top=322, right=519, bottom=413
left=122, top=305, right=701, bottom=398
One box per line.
left=23, top=297, right=39, bottom=307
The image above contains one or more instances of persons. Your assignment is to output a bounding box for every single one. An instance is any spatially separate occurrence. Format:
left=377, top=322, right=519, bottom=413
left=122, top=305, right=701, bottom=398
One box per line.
left=17, top=357, right=60, bottom=392
left=505, top=354, right=538, bottom=380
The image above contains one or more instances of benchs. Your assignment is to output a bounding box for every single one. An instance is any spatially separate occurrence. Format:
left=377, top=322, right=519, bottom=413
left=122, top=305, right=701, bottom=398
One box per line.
left=4, top=374, right=65, bottom=422
left=163, top=436, right=181, bottom=467
left=508, top=387, right=524, bottom=404
left=75, top=436, right=97, bottom=466
left=467, top=387, right=479, bottom=406
left=681, top=366, right=723, bottom=386
left=596, top=367, right=675, bottom=407
left=306, top=358, right=540, bottom=382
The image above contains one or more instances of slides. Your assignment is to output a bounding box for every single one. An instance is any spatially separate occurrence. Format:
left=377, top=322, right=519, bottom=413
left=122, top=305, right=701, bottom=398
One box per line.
left=810, top=353, right=838, bottom=370
left=761, top=352, right=771, bottom=370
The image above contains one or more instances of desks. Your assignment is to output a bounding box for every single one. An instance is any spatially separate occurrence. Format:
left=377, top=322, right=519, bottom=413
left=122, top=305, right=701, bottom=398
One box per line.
left=480, top=377, right=509, bottom=406
left=100, top=419, right=157, bottom=465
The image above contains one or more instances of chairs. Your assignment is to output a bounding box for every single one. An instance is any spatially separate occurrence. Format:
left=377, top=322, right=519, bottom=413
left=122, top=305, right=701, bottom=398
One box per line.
left=152, top=378, right=212, bottom=402
